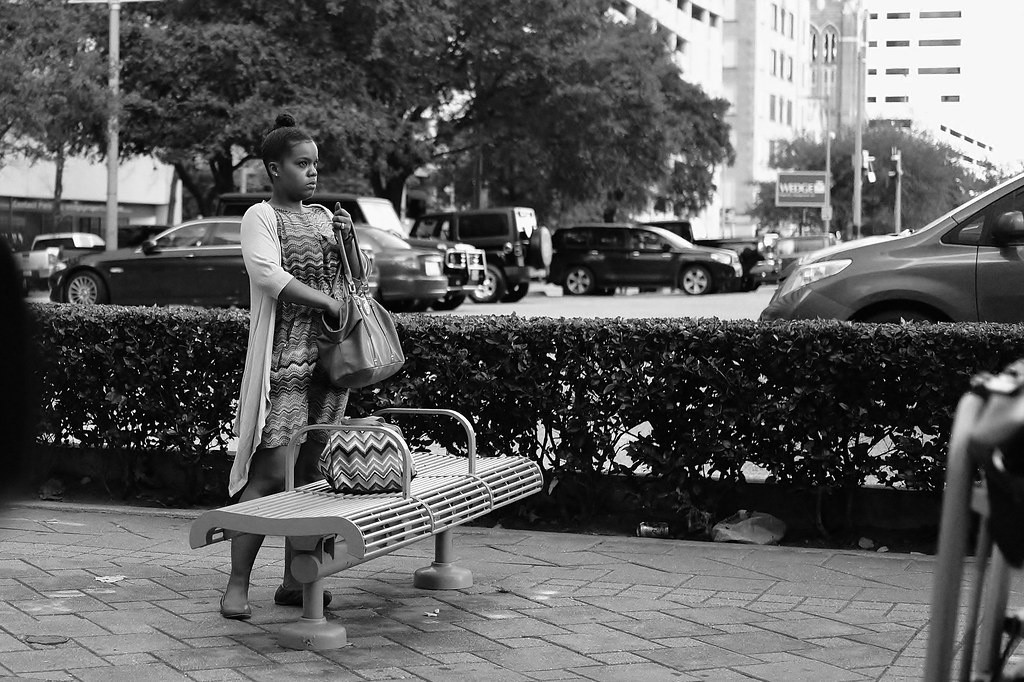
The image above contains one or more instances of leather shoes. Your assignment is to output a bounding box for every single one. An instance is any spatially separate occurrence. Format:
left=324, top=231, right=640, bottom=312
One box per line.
left=220, top=593, right=251, bottom=618
left=274, top=585, right=333, bottom=609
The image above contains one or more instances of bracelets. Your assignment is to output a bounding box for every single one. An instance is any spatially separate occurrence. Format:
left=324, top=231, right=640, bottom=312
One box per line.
left=335, top=229, right=353, bottom=243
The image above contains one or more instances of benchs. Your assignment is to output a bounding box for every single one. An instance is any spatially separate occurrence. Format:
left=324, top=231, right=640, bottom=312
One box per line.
left=189, top=451, right=543, bottom=651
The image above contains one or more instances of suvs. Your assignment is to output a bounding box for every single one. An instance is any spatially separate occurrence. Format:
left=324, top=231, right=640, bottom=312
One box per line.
left=409, top=207, right=553, bottom=305
left=217, top=189, right=490, bottom=313
left=546, top=223, right=744, bottom=296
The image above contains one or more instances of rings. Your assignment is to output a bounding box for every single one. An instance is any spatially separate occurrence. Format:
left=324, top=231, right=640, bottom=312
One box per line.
left=341, top=222, right=344, bottom=230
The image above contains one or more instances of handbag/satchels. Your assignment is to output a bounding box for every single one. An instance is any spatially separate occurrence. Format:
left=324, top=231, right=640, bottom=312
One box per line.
left=317, top=416, right=417, bottom=493
left=315, top=218, right=406, bottom=389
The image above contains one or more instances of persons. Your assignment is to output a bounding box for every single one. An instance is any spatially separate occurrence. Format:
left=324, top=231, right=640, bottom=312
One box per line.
left=220, top=114, right=371, bottom=619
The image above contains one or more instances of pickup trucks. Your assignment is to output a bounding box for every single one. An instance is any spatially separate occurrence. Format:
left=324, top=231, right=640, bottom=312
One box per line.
left=640, top=221, right=782, bottom=290
left=772, top=233, right=836, bottom=278
left=11, top=232, right=106, bottom=298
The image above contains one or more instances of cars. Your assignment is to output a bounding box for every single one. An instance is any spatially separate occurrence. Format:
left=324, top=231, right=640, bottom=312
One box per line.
left=352, top=223, right=448, bottom=307
left=759, top=170, right=1024, bottom=323
left=47, top=216, right=250, bottom=311
